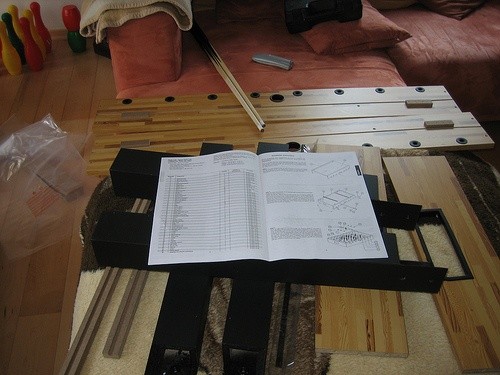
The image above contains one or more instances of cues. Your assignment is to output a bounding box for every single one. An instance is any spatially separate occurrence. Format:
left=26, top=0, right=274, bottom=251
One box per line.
left=191, top=21, right=265, bottom=134
left=193, top=20, right=266, bottom=128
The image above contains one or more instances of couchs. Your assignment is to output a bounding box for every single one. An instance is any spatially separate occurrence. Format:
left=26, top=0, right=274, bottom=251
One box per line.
left=106, top=0, right=500, bottom=123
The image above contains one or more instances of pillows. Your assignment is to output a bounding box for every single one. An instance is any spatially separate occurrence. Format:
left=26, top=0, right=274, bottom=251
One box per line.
left=420, top=0, right=488, bottom=21
left=300, top=0, right=414, bottom=55
